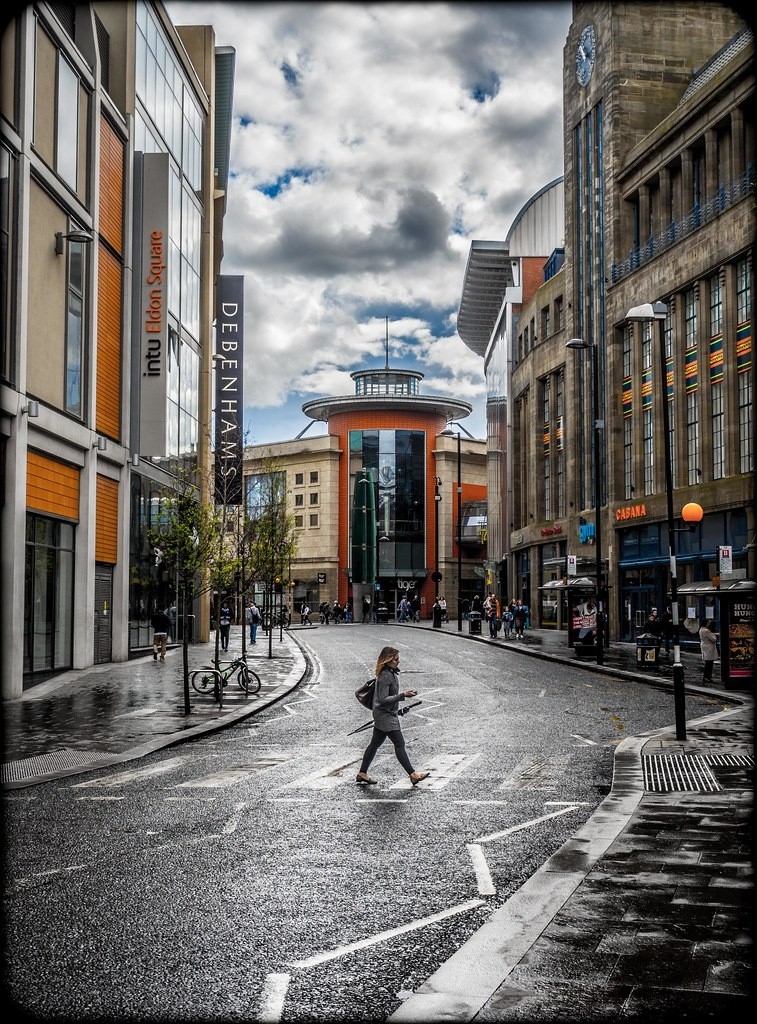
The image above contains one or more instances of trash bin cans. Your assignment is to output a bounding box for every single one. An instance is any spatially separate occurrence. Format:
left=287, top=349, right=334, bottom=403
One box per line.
left=636, top=632, right=661, bottom=672
left=378, top=607, right=388, bottom=623
left=468, top=611, right=482, bottom=635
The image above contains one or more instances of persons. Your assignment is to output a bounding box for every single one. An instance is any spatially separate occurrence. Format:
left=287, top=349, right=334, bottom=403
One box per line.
left=699, top=619, right=721, bottom=684
left=644, top=606, right=673, bottom=658
left=483, top=593, right=501, bottom=638
left=357, top=647, right=429, bottom=785
left=500, top=605, right=513, bottom=641
left=298, top=600, right=353, bottom=625
left=509, top=599, right=529, bottom=639
left=361, top=600, right=370, bottom=623
left=220, top=602, right=234, bottom=652
left=463, top=598, right=470, bottom=620
left=438, top=596, right=447, bottom=619
left=151, top=609, right=172, bottom=663
left=397, top=594, right=422, bottom=623
left=471, top=594, right=485, bottom=619
left=246, top=601, right=262, bottom=643
left=574, top=598, right=597, bottom=638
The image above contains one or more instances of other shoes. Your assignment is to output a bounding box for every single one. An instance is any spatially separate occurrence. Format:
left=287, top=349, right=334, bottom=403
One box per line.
left=154, top=652, right=157, bottom=660
left=505, top=635, right=507, bottom=638
left=410, top=773, right=430, bottom=786
left=516, top=634, right=519, bottom=637
left=494, top=635, right=497, bottom=639
left=225, top=649, right=228, bottom=651
left=356, top=775, right=378, bottom=785
left=251, top=640, right=256, bottom=643
left=490, top=634, right=493, bottom=638
left=507, top=635, right=510, bottom=638
left=222, top=645, right=224, bottom=649
left=703, top=678, right=713, bottom=684
left=160, top=657, right=164, bottom=661
left=521, top=635, right=523, bottom=638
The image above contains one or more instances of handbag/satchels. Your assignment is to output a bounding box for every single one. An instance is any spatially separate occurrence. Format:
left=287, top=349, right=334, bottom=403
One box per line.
left=496, top=618, right=502, bottom=631
left=354, top=679, right=373, bottom=710
left=249, top=608, right=259, bottom=624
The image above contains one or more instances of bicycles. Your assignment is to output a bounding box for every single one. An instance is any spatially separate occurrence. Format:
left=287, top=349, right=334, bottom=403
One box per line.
left=191, top=654, right=261, bottom=694
left=276, top=609, right=289, bottom=628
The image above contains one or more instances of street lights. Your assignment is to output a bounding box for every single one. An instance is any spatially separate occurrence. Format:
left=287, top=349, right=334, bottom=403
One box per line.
left=625, top=302, right=704, bottom=741
left=358, top=478, right=380, bottom=623
left=440, top=428, right=462, bottom=631
left=565, top=337, right=604, bottom=666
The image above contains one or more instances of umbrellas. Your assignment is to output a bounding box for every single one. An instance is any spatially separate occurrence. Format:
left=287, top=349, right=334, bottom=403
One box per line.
left=347, top=701, right=422, bottom=736
